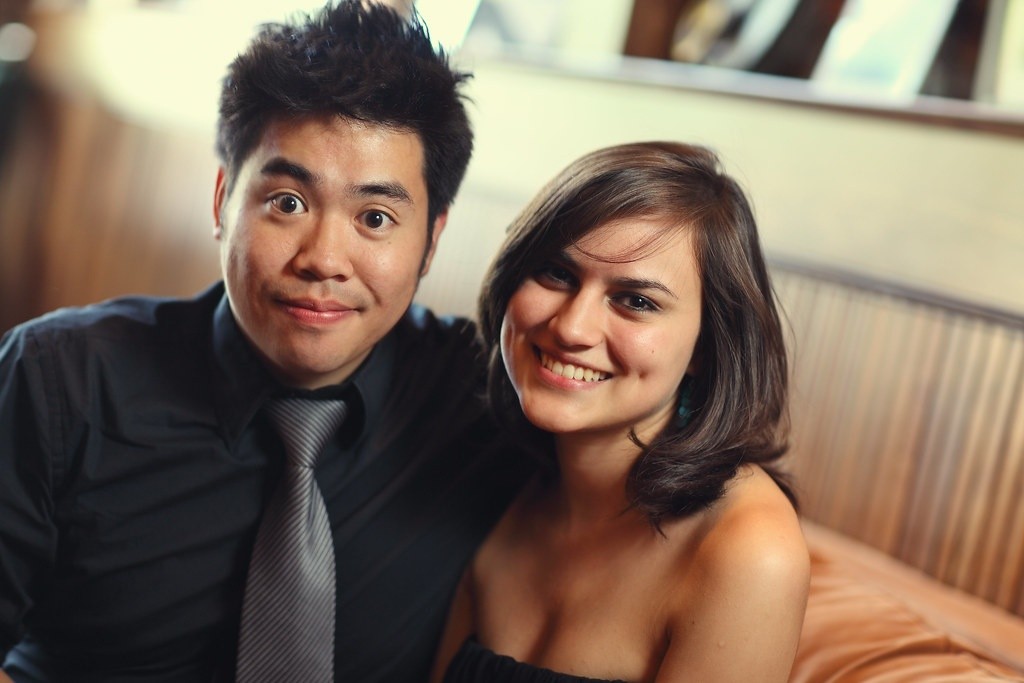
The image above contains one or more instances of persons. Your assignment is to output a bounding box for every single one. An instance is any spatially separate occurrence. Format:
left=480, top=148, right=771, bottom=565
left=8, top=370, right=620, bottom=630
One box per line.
left=0, top=2, right=491, bottom=683
left=425, top=139, right=809, bottom=683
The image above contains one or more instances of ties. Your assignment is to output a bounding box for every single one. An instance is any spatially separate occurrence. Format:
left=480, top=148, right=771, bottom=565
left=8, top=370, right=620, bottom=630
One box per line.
left=235, top=395, right=364, bottom=683
